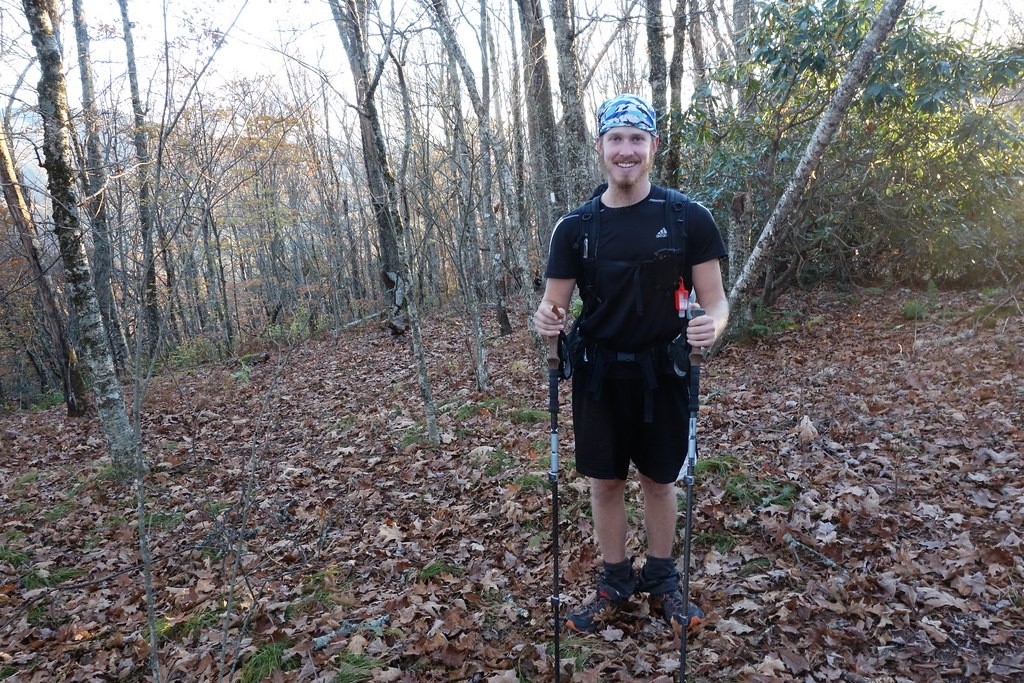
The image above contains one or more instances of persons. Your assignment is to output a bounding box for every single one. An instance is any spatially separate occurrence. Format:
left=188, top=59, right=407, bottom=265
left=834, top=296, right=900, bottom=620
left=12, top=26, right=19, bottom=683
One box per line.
left=532, top=93, right=730, bottom=634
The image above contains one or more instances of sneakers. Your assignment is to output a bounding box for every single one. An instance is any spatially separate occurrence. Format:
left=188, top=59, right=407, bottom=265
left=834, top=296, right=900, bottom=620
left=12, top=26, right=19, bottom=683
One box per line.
left=566, top=569, right=637, bottom=633
left=638, top=563, right=704, bottom=626
